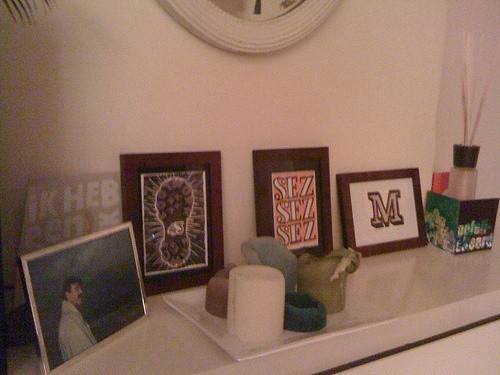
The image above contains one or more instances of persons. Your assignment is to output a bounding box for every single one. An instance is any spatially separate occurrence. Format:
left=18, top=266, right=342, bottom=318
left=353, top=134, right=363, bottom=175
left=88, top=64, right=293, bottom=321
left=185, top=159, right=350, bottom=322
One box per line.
left=57, top=276, right=97, bottom=362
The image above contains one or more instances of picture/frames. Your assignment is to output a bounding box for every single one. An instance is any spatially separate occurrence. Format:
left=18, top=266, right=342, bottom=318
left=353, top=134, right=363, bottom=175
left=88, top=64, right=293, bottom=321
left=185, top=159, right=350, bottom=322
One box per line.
left=120, top=150, right=225, bottom=297
left=336, top=168, right=428, bottom=258
left=18, top=220, right=151, bottom=375
left=252, top=146, right=334, bottom=258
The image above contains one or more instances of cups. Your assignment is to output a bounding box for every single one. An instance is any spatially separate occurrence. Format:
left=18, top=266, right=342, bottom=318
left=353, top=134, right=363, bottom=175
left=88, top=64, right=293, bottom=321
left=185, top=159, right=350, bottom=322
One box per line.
left=296, top=254, right=346, bottom=314
left=228, top=266, right=284, bottom=344
